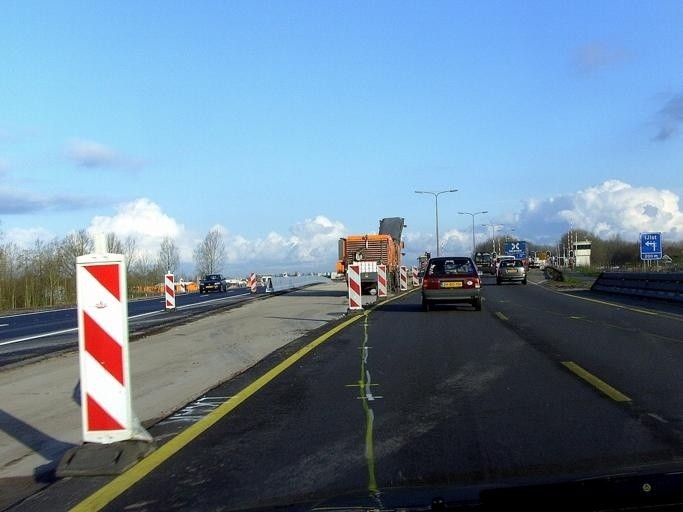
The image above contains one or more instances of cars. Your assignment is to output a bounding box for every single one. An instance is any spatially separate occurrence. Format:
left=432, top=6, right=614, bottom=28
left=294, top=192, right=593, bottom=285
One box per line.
left=420, top=256, right=481, bottom=312
left=198, top=274, right=226, bottom=294
left=496, top=259, right=527, bottom=285
left=474, top=252, right=515, bottom=274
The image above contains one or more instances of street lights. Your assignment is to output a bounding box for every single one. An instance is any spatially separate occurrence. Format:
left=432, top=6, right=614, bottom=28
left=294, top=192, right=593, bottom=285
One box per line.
left=414, top=190, right=458, bottom=258
left=481, top=224, right=503, bottom=252
left=457, top=211, right=488, bottom=255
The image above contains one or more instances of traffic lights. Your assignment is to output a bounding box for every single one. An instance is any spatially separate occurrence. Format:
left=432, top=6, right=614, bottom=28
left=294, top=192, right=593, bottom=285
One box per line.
left=492, top=252, right=496, bottom=258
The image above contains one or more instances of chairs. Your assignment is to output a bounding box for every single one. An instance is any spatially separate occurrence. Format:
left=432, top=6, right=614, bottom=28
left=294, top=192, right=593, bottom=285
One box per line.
left=433, top=265, right=445, bottom=273
